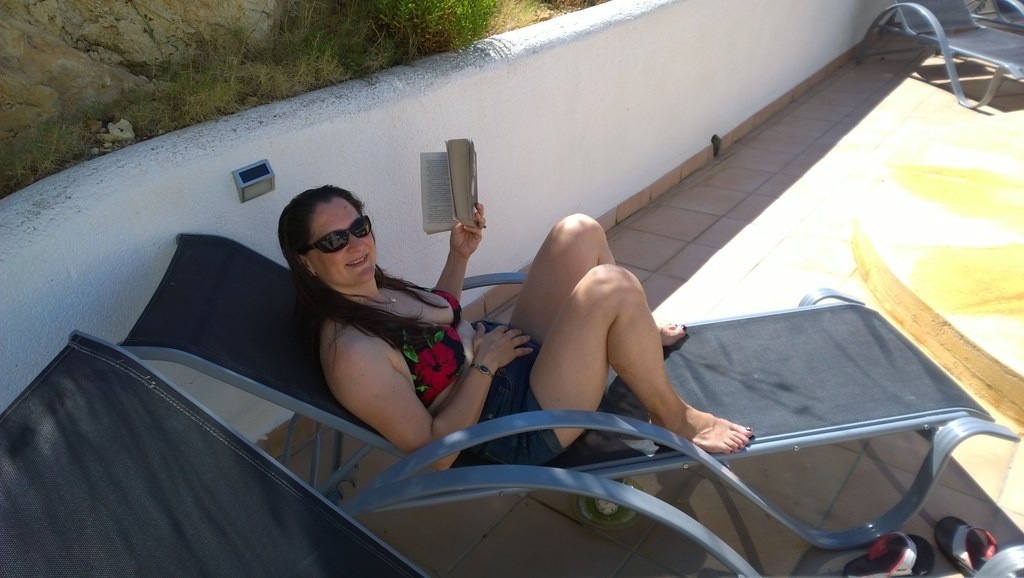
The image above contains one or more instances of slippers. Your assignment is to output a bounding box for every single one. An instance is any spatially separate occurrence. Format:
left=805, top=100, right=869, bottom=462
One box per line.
left=935, top=515, right=999, bottom=578
left=843, top=532, right=933, bottom=578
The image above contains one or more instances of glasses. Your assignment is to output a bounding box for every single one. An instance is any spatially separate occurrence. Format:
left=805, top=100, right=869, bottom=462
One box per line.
left=297, top=215, right=372, bottom=255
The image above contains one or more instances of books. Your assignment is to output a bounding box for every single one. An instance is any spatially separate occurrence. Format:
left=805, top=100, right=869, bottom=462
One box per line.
left=420, top=139, right=478, bottom=234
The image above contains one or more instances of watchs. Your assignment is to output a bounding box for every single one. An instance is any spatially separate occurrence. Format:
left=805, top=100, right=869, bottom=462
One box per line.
left=470, top=359, right=493, bottom=378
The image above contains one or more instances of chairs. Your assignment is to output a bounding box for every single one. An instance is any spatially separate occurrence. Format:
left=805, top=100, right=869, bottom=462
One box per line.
left=115, top=228, right=1024, bottom=553
left=856, top=0, right=1024, bottom=109
left=0, top=328, right=1024, bottom=578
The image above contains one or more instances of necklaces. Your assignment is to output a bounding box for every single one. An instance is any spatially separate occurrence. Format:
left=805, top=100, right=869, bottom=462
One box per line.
left=381, top=293, right=396, bottom=302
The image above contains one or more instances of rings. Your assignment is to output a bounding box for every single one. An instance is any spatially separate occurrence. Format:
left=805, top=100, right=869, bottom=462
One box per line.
left=482, top=226, right=486, bottom=228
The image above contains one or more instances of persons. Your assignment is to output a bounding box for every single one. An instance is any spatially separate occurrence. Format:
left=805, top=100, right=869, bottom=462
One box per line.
left=277, top=185, right=753, bottom=471
